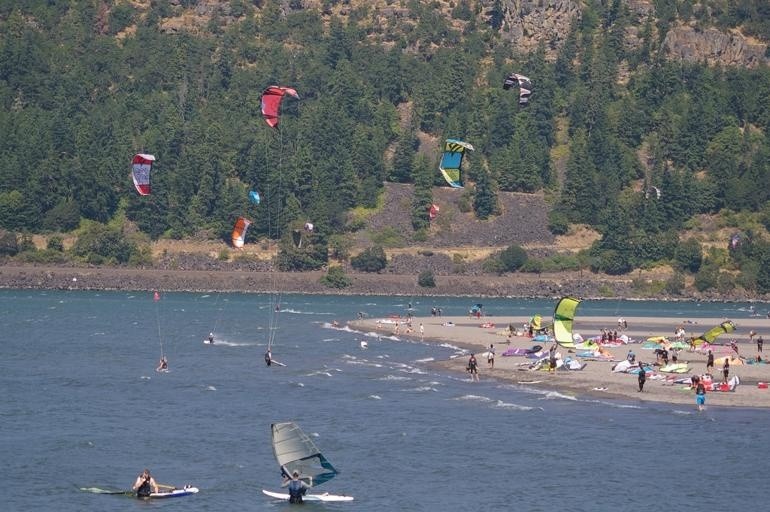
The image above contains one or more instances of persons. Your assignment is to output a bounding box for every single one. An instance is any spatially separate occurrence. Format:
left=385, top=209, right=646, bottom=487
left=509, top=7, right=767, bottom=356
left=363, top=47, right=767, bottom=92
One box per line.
left=332, top=301, right=770, bottom=413
left=208, top=333, right=216, bottom=344
left=132, top=468, right=158, bottom=498
left=265, top=350, right=272, bottom=367
left=158, top=360, right=167, bottom=370
left=280, top=471, right=313, bottom=505
left=275, top=304, right=280, bottom=312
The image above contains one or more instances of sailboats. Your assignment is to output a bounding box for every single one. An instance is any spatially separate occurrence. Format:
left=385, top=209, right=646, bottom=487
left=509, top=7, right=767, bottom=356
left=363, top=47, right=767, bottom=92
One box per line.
left=259, top=420, right=357, bottom=505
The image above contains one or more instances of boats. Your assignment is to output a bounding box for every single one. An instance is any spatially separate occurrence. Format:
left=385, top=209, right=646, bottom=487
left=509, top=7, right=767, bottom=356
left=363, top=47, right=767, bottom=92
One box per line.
left=151, top=484, right=200, bottom=502
